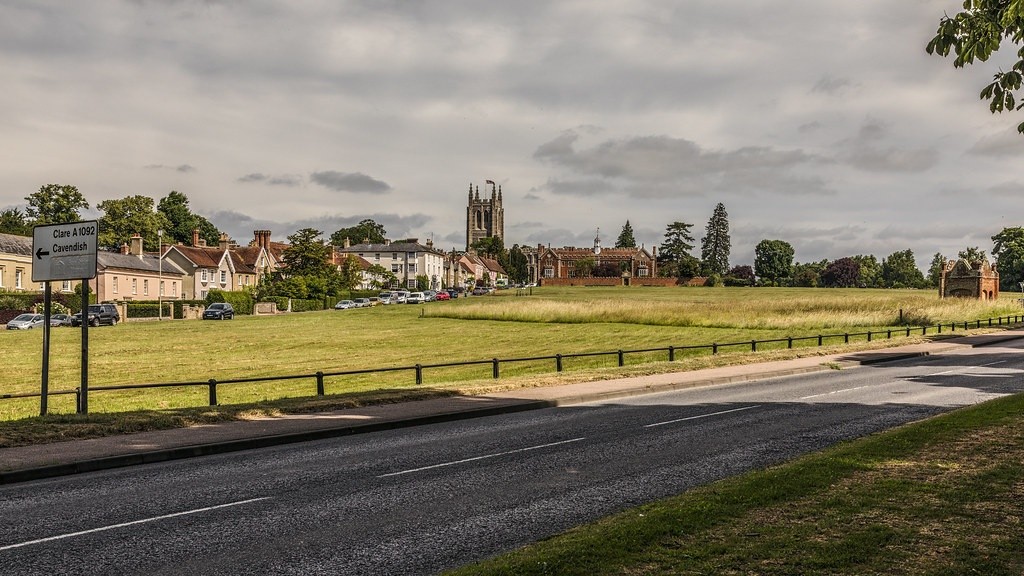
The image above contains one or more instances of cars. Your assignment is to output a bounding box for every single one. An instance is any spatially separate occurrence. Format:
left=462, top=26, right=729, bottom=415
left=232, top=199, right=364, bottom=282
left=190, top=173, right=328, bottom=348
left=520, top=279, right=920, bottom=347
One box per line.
left=6, top=304, right=45, bottom=330
left=336, top=286, right=495, bottom=309
left=50, top=313, right=72, bottom=327
left=203, top=303, right=235, bottom=320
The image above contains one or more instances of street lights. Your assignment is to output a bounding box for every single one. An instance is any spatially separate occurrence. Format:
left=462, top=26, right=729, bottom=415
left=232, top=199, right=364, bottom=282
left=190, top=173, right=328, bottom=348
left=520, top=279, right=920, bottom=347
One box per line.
left=158, top=229, right=163, bottom=320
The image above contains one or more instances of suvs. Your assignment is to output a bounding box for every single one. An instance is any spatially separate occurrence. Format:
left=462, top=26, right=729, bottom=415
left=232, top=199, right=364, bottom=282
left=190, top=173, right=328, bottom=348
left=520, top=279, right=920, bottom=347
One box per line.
left=72, top=304, right=120, bottom=327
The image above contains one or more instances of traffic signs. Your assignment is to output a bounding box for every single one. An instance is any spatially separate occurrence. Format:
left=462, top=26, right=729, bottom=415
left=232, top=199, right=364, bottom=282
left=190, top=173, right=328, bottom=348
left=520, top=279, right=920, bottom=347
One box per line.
left=32, top=221, right=98, bottom=282
